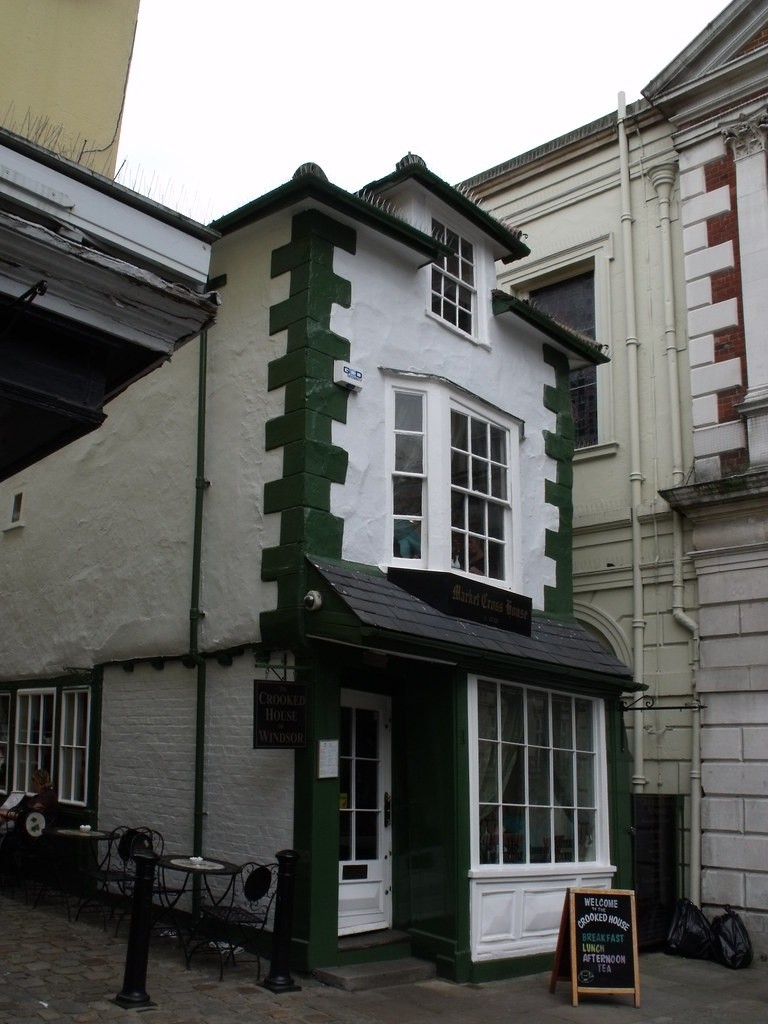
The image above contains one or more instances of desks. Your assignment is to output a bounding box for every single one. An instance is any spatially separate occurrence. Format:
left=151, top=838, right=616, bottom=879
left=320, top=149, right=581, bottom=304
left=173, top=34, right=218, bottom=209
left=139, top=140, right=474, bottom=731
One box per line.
left=154, top=854, right=243, bottom=970
left=41, top=827, right=120, bottom=922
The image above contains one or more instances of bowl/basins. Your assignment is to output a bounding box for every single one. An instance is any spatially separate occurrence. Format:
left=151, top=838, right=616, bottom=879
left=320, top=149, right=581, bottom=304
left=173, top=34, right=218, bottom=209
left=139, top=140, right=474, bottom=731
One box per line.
left=80, top=826, right=91, bottom=832
left=190, top=857, right=204, bottom=865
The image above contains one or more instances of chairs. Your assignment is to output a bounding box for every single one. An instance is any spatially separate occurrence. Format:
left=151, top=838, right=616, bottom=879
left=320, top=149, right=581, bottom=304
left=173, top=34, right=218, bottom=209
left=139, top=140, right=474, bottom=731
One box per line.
left=185, top=861, right=283, bottom=982
left=0, top=806, right=65, bottom=908
left=74, top=826, right=183, bottom=946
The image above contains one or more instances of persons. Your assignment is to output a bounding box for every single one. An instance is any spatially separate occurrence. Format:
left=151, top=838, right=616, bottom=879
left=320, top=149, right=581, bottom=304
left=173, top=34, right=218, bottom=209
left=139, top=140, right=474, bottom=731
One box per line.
left=2, top=769, right=55, bottom=891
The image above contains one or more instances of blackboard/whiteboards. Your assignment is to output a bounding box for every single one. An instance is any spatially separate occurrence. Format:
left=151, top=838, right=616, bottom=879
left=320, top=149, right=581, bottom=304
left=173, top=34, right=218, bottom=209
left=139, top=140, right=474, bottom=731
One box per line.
left=568, top=886, right=641, bottom=994
